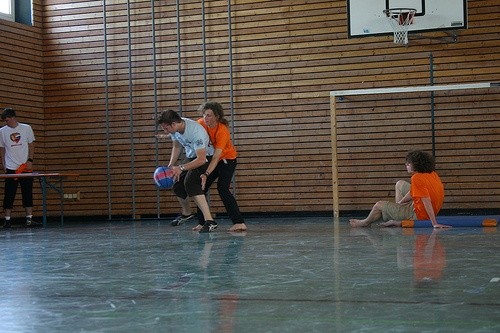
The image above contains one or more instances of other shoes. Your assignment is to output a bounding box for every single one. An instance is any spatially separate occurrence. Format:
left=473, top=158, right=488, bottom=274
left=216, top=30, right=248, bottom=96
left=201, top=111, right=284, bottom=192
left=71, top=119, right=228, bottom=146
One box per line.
left=3, top=220, right=11, bottom=229
left=26, top=216, right=32, bottom=227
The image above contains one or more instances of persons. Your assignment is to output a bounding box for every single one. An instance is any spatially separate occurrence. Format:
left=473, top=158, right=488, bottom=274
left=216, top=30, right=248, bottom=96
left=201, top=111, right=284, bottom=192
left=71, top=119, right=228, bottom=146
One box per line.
left=192, top=102, right=247, bottom=231
left=157, top=109, right=218, bottom=233
left=350, top=152, right=451, bottom=228
left=351, top=228, right=448, bottom=294
left=0, top=108, right=43, bottom=229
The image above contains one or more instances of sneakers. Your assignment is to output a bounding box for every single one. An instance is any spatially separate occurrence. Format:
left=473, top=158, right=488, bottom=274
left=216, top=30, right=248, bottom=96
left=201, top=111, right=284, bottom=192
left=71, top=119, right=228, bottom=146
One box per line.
left=199, top=221, right=217, bottom=233
left=171, top=214, right=193, bottom=226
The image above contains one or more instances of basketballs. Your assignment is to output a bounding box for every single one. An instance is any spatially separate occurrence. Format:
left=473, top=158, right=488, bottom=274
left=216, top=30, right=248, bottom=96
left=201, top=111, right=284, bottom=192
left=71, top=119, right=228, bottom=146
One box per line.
left=153, top=167, right=175, bottom=189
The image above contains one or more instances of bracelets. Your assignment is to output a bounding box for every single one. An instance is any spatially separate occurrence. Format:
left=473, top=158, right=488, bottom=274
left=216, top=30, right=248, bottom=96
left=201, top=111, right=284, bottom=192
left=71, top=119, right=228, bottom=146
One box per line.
left=179, top=165, right=184, bottom=171
left=28, top=159, right=33, bottom=162
left=204, top=171, right=209, bottom=177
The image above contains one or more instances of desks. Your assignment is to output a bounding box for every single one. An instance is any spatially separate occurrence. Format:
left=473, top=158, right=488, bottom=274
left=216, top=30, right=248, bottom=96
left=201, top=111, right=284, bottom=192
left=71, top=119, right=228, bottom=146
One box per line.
left=0, top=172, right=80, bottom=224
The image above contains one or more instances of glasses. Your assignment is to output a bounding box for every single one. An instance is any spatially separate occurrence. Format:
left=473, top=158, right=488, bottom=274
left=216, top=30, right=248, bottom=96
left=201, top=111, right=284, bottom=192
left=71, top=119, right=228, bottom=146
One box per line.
left=405, top=162, right=410, bottom=166
